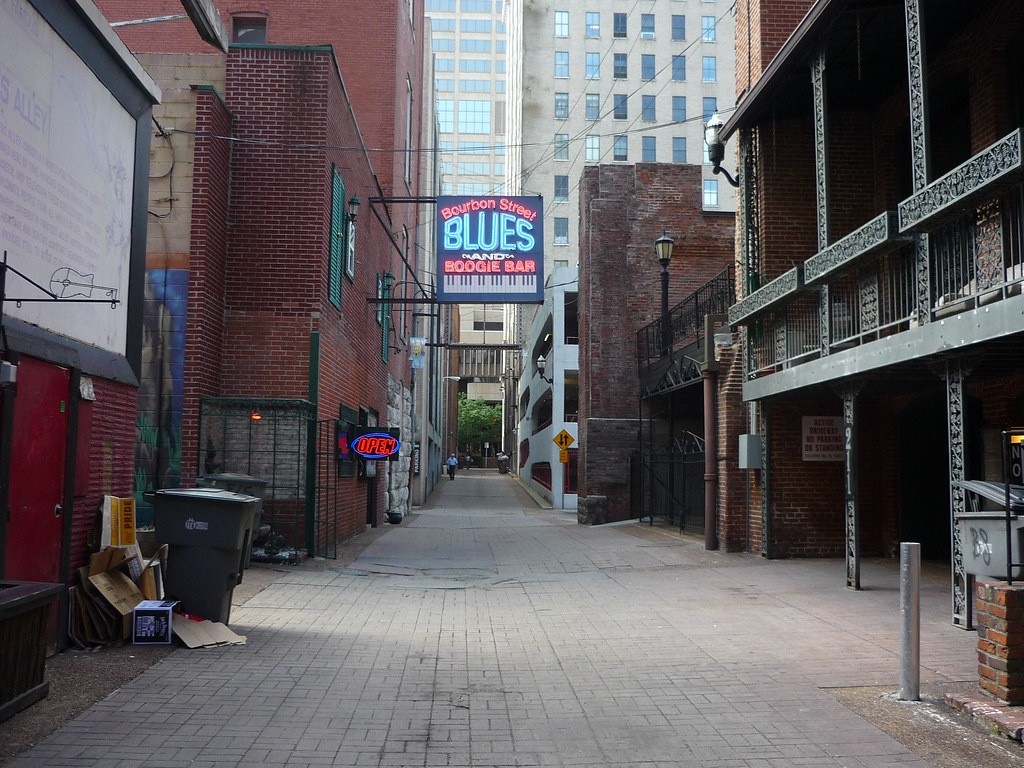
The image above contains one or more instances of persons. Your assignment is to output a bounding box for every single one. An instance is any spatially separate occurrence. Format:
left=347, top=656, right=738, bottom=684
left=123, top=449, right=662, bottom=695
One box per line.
left=446, top=453, right=458, bottom=481
left=466, top=455, right=471, bottom=470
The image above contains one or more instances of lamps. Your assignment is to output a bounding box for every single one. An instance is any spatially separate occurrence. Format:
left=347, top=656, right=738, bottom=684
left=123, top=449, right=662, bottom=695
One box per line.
left=536, top=355, right=554, bottom=384
left=702, top=108, right=739, bottom=187
left=382, top=269, right=396, bottom=290
left=344, top=192, right=360, bottom=224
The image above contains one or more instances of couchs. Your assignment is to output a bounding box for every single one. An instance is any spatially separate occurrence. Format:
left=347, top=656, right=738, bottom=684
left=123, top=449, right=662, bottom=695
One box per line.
left=909, top=262, right=1024, bottom=331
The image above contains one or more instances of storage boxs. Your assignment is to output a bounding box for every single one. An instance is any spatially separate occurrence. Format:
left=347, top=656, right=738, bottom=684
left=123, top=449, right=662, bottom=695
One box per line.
left=133, top=600, right=181, bottom=644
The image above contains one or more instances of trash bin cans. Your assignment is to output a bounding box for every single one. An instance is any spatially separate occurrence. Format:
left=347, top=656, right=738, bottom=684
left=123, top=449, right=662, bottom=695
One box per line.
left=143, top=486, right=262, bottom=627
left=202, top=473, right=270, bottom=570
left=952, top=479, right=1024, bottom=583
left=498, top=460, right=508, bottom=474
